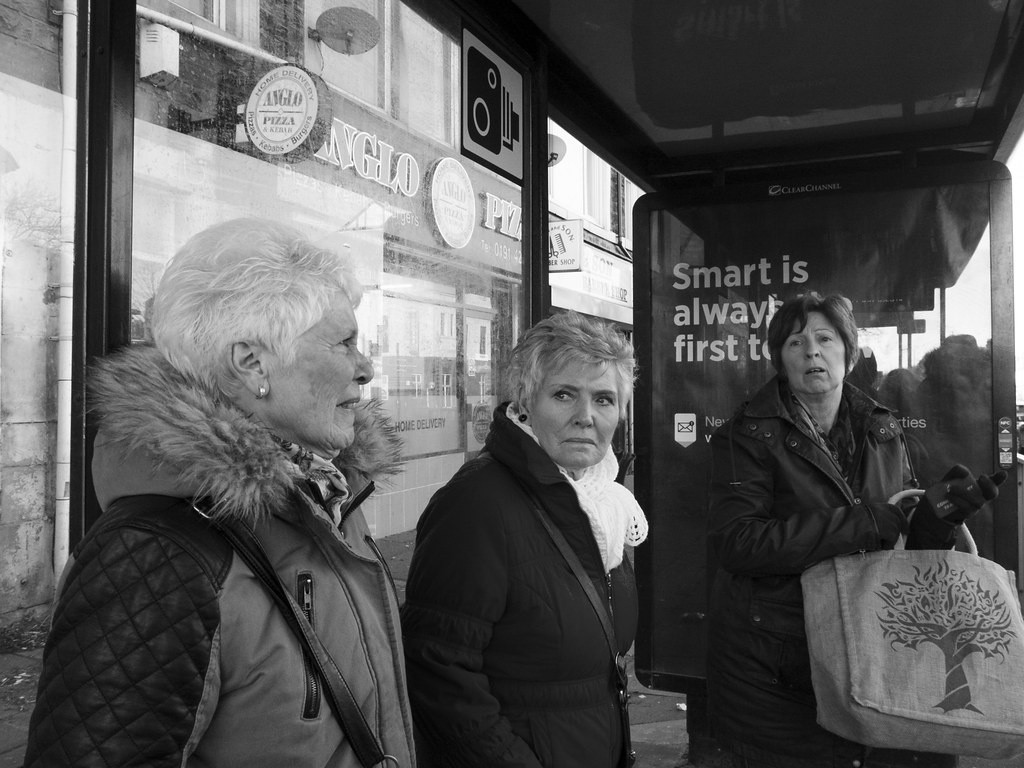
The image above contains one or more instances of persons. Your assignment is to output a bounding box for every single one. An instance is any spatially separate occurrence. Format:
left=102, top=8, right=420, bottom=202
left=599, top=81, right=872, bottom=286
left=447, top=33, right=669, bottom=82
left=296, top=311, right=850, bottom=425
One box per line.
left=705, top=292, right=994, bottom=768
left=23, top=216, right=414, bottom=768
left=402, top=312, right=643, bottom=768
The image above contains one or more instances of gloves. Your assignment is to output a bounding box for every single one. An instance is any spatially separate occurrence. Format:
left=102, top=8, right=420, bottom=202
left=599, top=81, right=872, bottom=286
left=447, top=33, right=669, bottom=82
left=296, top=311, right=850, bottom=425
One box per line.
left=924, top=463, right=1008, bottom=525
left=865, top=502, right=912, bottom=551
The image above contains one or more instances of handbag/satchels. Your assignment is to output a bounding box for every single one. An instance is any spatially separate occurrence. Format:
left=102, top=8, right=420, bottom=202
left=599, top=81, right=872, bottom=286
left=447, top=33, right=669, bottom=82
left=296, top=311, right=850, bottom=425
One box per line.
left=798, top=487, right=1024, bottom=761
